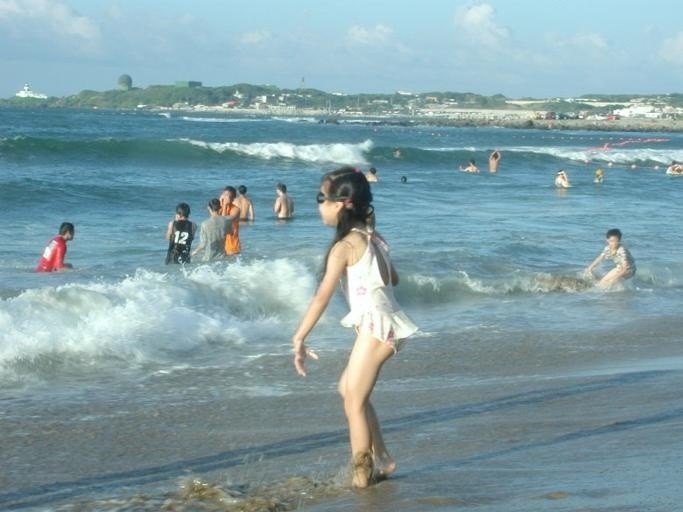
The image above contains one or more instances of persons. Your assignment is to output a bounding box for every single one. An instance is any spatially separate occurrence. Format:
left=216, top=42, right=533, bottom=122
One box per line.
left=292, top=167, right=421, bottom=489
left=218, top=188, right=242, bottom=254
left=553, top=170, right=574, bottom=188
left=366, top=168, right=378, bottom=183
left=400, top=176, right=406, bottom=182
left=666, top=161, right=683, bottom=175
left=393, top=148, right=401, bottom=159
left=234, top=187, right=253, bottom=222
left=593, top=169, right=604, bottom=184
left=274, top=184, right=293, bottom=221
left=457, top=159, right=481, bottom=174
left=33, top=222, right=74, bottom=271
left=164, top=203, right=197, bottom=264
left=192, top=201, right=233, bottom=262
left=587, top=229, right=637, bottom=289
left=489, top=149, right=501, bottom=173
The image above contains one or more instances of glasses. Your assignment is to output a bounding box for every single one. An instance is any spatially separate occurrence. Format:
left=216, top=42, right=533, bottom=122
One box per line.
left=316, top=194, right=349, bottom=203
